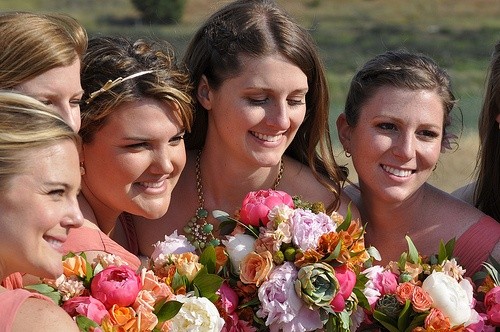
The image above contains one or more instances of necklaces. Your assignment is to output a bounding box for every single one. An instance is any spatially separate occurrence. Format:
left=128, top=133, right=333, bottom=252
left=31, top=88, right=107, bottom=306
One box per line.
left=192, top=153, right=286, bottom=250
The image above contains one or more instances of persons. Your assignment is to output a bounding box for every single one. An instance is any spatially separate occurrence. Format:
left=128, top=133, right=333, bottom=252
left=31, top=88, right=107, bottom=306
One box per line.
left=134, top=0, right=364, bottom=262
left=59, top=32, right=196, bottom=280
left=0, top=91, right=86, bottom=332
left=0, top=12, right=90, bottom=133
left=447, top=41, right=500, bottom=230
left=335, top=50, right=500, bottom=272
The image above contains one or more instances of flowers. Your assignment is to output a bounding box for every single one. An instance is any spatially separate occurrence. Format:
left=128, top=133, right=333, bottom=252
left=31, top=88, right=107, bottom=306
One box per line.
left=20, top=188, right=500, bottom=332
